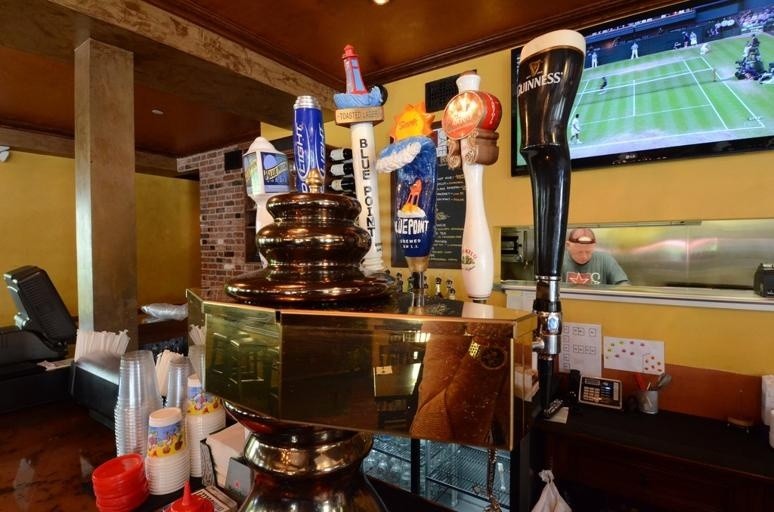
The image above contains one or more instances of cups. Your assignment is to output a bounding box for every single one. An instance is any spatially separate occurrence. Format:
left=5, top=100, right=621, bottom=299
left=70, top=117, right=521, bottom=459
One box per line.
left=364, top=435, right=430, bottom=496
left=115, top=334, right=225, bottom=496
left=639, top=387, right=658, bottom=415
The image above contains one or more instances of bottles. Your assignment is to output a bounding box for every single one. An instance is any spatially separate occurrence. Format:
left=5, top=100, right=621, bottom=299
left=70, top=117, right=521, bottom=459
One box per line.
left=386, top=269, right=456, bottom=301
left=160, top=480, right=214, bottom=511
left=326, top=147, right=355, bottom=196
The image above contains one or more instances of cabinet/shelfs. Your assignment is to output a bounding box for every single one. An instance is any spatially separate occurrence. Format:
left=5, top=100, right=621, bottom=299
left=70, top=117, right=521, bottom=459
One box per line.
left=527, top=384, right=774, bottom=512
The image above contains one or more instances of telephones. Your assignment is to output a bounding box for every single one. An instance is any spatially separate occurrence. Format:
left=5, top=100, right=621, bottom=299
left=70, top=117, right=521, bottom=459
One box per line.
left=567, top=369, right=622, bottom=413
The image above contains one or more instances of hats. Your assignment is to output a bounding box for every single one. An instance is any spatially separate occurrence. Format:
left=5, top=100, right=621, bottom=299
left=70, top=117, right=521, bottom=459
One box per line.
left=567, top=228, right=598, bottom=245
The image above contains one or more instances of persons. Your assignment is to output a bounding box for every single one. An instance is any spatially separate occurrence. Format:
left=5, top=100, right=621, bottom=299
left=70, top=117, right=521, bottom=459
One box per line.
left=596, top=76, right=608, bottom=96
left=409, top=321, right=512, bottom=444
left=558, top=224, right=634, bottom=288
left=672, top=6, right=774, bottom=84
left=569, top=111, right=585, bottom=145
left=590, top=50, right=598, bottom=68
left=629, top=42, right=640, bottom=59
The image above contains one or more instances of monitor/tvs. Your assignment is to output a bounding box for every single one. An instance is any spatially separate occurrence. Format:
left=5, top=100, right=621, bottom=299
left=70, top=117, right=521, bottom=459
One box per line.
left=511, top=0, right=774, bottom=177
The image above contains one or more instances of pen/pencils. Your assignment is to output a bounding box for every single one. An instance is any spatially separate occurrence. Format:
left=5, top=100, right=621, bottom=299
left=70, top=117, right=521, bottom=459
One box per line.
left=634, top=371, right=672, bottom=410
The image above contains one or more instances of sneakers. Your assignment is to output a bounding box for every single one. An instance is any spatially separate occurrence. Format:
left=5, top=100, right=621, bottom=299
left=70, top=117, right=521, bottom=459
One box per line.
left=567, top=139, right=582, bottom=146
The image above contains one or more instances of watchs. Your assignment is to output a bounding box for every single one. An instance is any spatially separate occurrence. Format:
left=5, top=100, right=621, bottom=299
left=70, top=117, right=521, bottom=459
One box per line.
left=467, top=341, right=508, bottom=370
